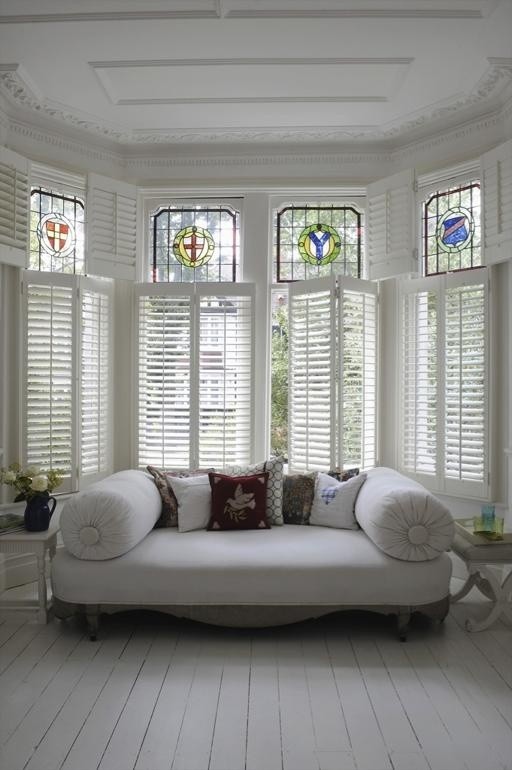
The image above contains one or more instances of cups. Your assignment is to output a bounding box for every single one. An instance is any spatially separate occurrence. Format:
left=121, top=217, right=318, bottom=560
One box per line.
left=480, top=503, right=496, bottom=535
left=487, top=517, right=503, bottom=540
left=473, top=516, right=485, bottom=534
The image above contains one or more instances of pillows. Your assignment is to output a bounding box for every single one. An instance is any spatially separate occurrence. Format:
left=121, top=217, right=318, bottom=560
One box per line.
left=141, top=463, right=369, bottom=531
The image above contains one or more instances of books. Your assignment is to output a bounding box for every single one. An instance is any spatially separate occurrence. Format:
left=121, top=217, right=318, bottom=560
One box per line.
left=0, top=513, right=24, bottom=536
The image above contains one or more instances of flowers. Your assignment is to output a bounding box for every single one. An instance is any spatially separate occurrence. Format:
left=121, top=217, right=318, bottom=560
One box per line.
left=5, top=466, right=69, bottom=495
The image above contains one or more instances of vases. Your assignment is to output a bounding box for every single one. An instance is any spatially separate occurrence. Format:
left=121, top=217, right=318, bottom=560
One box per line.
left=13, top=493, right=60, bottom=531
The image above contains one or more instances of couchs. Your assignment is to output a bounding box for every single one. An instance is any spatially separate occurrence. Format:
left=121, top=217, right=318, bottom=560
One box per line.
left=49, top=458, right=454, bottom=645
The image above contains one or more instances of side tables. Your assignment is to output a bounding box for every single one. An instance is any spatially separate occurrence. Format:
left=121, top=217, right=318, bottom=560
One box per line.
left=450, top=517, right=512, bottom=636
left=0, top=525, right=59, bottom=626
